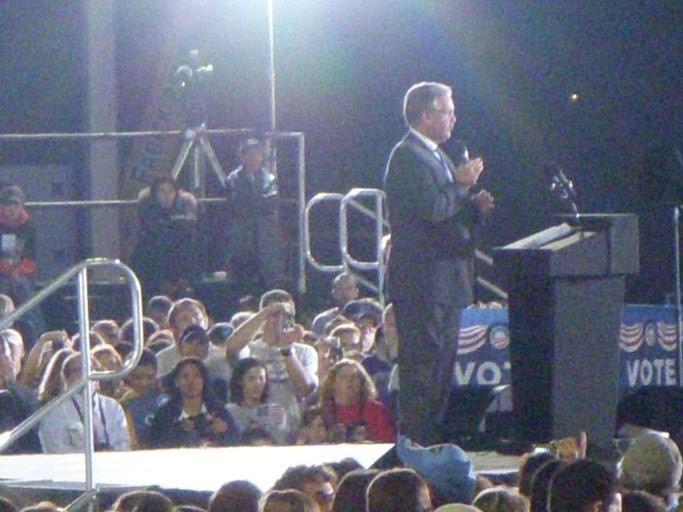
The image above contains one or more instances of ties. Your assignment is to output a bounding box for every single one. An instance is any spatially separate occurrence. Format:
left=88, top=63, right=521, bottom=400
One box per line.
left=434, top=149, right=453, bottom=185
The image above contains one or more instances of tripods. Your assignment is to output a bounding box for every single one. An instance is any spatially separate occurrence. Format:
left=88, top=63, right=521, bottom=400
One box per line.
left=167, top=124, right=226, bottom=197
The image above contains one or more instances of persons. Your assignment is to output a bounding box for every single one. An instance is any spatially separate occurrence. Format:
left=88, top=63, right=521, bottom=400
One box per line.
left=130, top=172, right=199, bottom=301
left=1, top=430, right=683, bottom=512
left=0, top=185, right=36, bottom=278
left=384, top=81, right=493, bottom=446
left=224, top=140, right=282, bottom=297
left=1, top=274, right=526, bottom=452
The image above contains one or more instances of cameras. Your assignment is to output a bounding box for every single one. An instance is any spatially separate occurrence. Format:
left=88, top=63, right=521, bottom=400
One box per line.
left=52, top=336, right=64, bottom=351
left=322, top=337, right=340, bottom=349
left=281, top=310, right=293, bottom=335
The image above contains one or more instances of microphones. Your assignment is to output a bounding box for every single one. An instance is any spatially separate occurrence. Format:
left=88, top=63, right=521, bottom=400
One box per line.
left=545, top=162, right=577, bottom=204
left=450, top=140, right=477, bottom=189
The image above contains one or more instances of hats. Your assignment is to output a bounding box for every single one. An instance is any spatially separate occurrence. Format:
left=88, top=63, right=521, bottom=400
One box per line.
left=622, top=434, right=683, bottom=487
left=0, top=186, right=23, bottom=203
left=345, top=300, right=382, bottom=326
left=396, top=435, right=476, bottom=503
